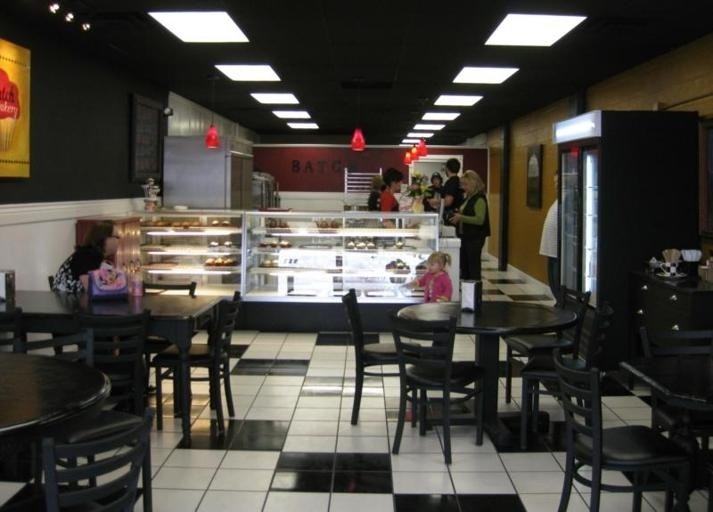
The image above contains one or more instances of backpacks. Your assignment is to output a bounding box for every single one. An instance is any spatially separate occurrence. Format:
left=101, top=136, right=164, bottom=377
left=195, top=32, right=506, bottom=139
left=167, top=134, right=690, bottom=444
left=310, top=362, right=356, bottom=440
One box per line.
left=52, top=256, right=83, bottom=292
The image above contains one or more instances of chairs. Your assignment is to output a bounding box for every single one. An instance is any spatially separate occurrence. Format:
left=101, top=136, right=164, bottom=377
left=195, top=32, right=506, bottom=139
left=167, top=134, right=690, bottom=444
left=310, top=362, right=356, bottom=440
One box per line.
left=152, top=291, right=242, bottom=430
left=390, top=311, right=485, bottom=464
left=505, top=286, right=590, bottom=402
left=522, top=303, right=613, bottom=445
left=343, top=288, right=421, bottom=427
left=0, top=406, right=154, bottom=512
left=552, top=349, right=687, bottom=512
left=73, top=308, right=150, bottom=448
left=640, top=327, right=713, bottom=449
left=142, top=281, right=197, bottom=417
left=14, top=328, right=93, bottom=486
left=50, top=277, right=90, bottom=355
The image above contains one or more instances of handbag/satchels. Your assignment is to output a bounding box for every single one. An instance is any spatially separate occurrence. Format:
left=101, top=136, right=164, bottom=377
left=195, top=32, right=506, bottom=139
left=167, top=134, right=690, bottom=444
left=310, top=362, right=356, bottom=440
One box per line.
left=87, top=267, right=128, bottom=296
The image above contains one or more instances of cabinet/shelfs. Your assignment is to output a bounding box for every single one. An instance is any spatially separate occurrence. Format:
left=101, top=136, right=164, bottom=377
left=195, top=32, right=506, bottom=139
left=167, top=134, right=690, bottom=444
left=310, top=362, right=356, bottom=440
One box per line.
left=631, top=269, right=713, bottom=331
left=244, top=210, right=438, bottom=332
left=131, top=210, right=243, bottom=330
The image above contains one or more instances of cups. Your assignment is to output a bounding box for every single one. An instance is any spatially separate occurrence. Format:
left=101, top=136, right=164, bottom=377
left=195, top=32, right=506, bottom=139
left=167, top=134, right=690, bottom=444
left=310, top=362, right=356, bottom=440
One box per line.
left=683, top=261, right=697, bottom=287
left=132, top=273, right=143, bottom=296
left=660, top=260, right=682, bottom=275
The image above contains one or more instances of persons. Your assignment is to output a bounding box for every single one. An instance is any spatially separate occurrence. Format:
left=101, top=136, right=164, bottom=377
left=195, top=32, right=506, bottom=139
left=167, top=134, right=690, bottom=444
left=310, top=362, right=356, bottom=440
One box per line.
left=52, top=220, right=158, bottom=396
left=448, top=169, right=491, bottom=281
left=423, top=171, right=444, bottom=211
left=380, top=168, right=419, bottom=229
left=538, top=170, right=560, bottom=309
left=427, top=157, right=463, bottom=211
left=367, top=174, right=384, bottom=209
left=404, top=251, right=453, bottom=347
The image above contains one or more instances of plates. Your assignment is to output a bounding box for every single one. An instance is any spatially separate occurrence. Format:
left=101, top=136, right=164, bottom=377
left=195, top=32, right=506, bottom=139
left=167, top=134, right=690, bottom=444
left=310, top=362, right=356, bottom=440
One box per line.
left=656, top=272, right=687, bottom=278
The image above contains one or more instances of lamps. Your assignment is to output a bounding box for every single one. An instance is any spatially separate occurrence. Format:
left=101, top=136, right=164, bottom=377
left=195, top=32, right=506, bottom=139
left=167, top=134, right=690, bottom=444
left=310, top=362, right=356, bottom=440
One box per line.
left=206, top=76, right=220, bottom=148
left=351, top=85, right=365, bottom=152
left=403, top=138, right=428, bottom=164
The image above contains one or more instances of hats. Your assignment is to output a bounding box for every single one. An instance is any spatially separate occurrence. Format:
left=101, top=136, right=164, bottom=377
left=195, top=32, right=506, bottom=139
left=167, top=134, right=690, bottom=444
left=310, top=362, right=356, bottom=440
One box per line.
left=432, top=172, right=441, bottom=178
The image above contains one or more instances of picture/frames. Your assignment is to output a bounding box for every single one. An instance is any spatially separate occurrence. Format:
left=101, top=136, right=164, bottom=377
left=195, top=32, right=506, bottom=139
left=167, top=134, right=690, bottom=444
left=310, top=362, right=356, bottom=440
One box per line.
left=128, top=95, right=166, bottom=181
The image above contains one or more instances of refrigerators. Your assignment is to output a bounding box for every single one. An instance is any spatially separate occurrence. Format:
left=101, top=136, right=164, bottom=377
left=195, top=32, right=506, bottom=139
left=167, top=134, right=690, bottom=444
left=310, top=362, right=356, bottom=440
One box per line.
left=163, top=135, right=255, bottom=209
left=552, top=110, right=700, bottom=362
